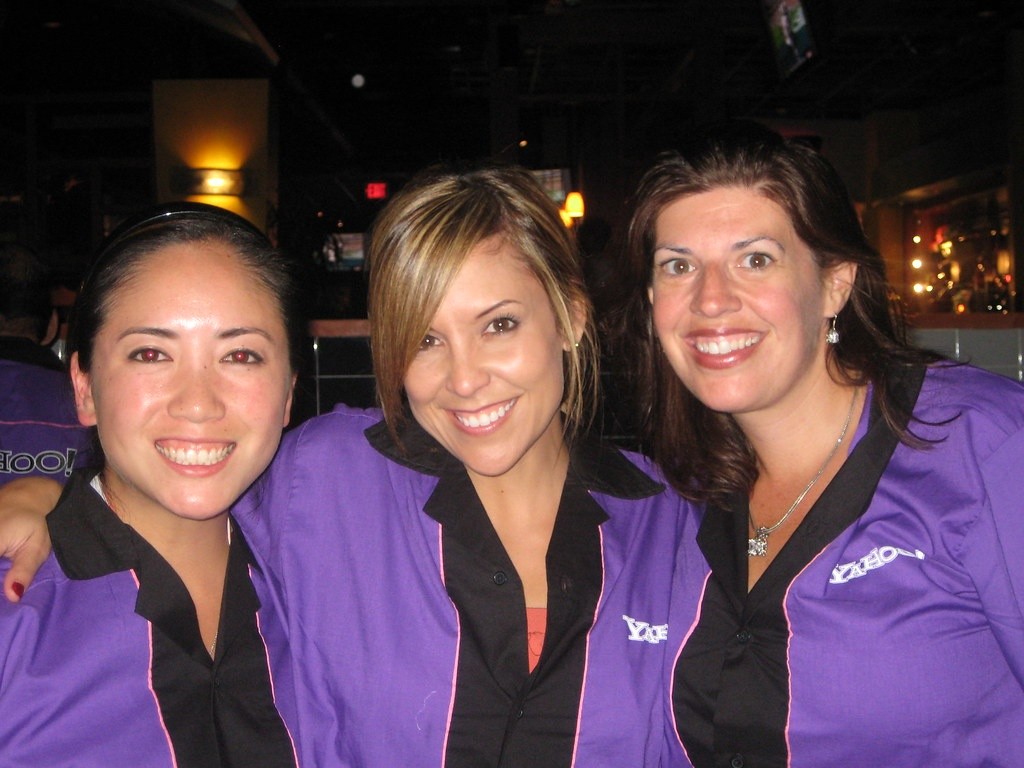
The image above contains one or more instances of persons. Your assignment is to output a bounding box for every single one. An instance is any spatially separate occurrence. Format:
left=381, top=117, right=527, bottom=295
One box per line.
left=0, top=246, right=83, bottom=493
left=599, top=126, right=1024, bottom=768
left=0, top=202, right=302, bottom=768
left=0, top=164, right=688, bottom=768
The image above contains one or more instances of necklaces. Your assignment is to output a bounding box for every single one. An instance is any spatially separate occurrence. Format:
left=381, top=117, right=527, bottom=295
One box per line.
left=747, top=382, right=859, bottom=558
